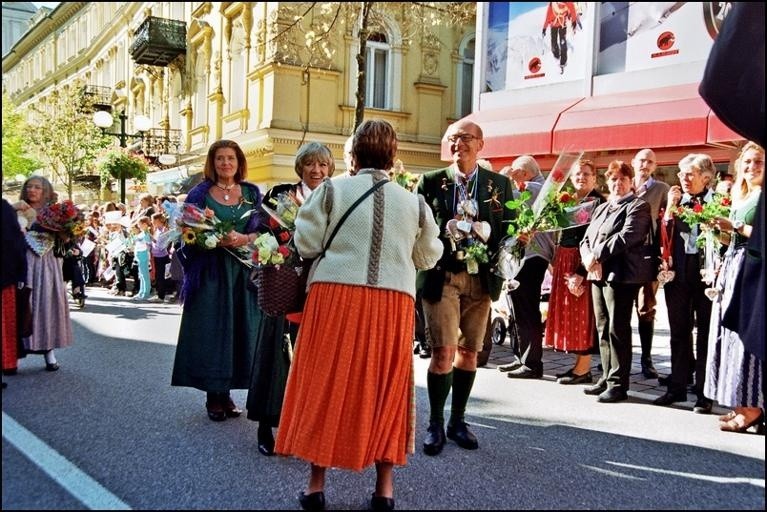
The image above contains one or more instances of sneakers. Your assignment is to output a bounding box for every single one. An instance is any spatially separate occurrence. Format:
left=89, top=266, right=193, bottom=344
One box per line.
left=147, top=294, right=164, bottom=303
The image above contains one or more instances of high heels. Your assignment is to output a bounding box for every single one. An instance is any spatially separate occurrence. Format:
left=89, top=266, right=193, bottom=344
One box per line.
left=257, top=428, right=279, bottom=455
left=720, top=407, right=764, bottom=433
left=44, top=348, right=60, bottom=371
left=719, top=410, right=738, bottom=422
left=222, top=396, right=241, bottom=418
left=206, top=396, right=226, bottom=420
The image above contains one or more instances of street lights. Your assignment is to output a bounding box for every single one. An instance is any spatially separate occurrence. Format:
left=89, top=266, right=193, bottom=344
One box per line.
left=94, top=105, right=152, bottom=204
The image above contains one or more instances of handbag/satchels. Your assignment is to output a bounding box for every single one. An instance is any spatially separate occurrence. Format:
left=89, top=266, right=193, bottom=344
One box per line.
left=257, top=236, right=310, bottom=316
left=625, top=197, right=661, bottom=283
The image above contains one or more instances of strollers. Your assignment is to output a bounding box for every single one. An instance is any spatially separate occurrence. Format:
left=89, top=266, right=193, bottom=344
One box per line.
left=489, top=276, right=552, bottom=353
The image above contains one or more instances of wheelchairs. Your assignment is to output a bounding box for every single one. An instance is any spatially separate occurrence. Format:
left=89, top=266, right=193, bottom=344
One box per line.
left=56, top=247, right=91, bottom=308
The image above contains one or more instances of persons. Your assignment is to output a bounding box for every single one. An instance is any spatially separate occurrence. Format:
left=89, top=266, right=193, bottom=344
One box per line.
left=541, top=0, right=577, bottom=76
left=1, top=199, right=28, bottom=388
left=246, top=141, right=337, bottom=455
left=545, top=158, right=607, bottom=384
left=157, top=194, right=187, bottom=215
left=414, top=119, right=530, bottom=454
left=149, top=214, right=169, bottom=302
left=497, top=156, right=558, bottom=379
left=131, top=216, right=153, bottom=302
left=655, top=154, right=732, bottom=412
left=138, top=194, right=155, bottom=219
left=699, top=1, right=767, bottom=367
left=413, top=288, right=432, bottom=359
left=476, top=158, right=495, bottom=366
left=64, top=242, right=88, bottom=300
left=707, top=142, right=765, bottom=433
left=628, top=149, right=672, bottom=378
left=85, top=201, right=138, bottom=295
left=579, top=159, right=652, bottom=400
left=171, top=141, right=263, bottom=423
left=275, top=117, right=444, bottom=511
left=16, top=176, right=71, bottom=371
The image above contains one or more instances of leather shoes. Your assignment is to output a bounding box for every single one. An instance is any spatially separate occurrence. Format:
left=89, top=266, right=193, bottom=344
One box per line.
left=447, top=422, right=478, bottom=449
left=373, top=491, right=394, bottom=511
left=695, top=397, right=712, bottom=414
left=424, top=421, right=445, bottom=454
left=507, top=364, right=544, bottom=379
left=642, top=364, right=660, bottom=378
left=656, top=391, right=687, bottom=405
left=418, top=344, right=432, bottom=358
left=596, top=389, right=628, bottom=402
left=660, top=374, right=694, bottom=386
left=557, top=372, right=592, bottom=384
left=499, top=361, right=522, bottom=372
left=584, top=383, right=607, bottom=394
left=299, top=488, right=326, bottom=511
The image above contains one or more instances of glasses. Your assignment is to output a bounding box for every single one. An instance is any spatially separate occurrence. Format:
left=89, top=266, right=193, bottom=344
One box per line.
left=678, top=171, right=700, bottom=180
left=449, top=133, right=483, bottom=144
